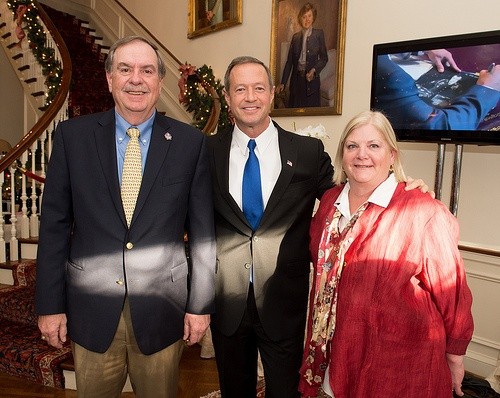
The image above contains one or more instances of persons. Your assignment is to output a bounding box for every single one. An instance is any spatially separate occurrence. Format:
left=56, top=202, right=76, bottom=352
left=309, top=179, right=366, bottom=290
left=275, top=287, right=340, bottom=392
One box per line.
left=35, top=34, right=217, bottom=398
left=210, top=55, right=436, bottom=398
left=378, top=48, right=500, bottom=130
left=294, top=111, right=473, bottom=398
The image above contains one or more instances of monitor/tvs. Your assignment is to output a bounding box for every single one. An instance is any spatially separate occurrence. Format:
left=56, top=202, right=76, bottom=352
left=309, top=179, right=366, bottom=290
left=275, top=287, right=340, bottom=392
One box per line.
left=369, top=30, right=500, bottom=145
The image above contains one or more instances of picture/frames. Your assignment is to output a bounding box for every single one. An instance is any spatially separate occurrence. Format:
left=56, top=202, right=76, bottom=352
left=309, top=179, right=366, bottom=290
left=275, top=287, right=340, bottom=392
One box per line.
left=187, top=0, right=243, bottom=39
left=270, top=0, right=347, bottom=117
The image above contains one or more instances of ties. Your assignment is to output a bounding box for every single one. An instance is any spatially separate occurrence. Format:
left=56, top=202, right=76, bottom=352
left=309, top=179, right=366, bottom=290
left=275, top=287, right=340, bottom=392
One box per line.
left=242, top=138, right=264, bottom=282
left=120, top=127, right=143, bottom=229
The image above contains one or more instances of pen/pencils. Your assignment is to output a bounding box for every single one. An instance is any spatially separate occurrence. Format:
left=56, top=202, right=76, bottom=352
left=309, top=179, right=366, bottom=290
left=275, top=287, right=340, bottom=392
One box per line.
left=488, top=63, right=495, bottom=72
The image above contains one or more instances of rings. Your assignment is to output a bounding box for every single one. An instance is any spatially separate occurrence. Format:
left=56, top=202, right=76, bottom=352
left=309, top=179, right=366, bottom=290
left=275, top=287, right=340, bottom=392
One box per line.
left=41, top=335, right=46, bottom=340
